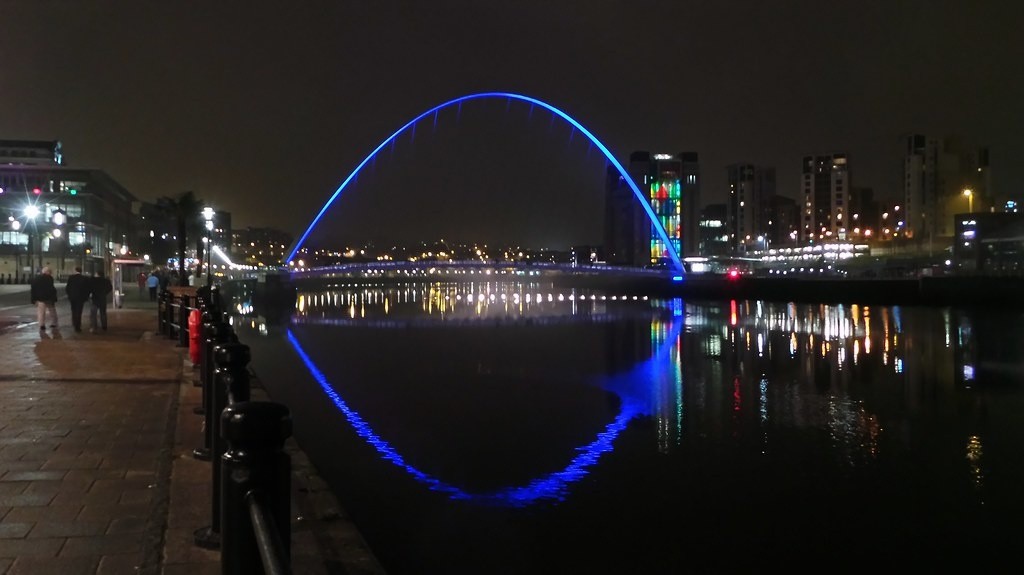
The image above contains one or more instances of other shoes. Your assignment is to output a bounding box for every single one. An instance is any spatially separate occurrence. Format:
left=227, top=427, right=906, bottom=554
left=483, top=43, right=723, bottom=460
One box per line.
left=51, top=324, right=58, bottom=327
left=90, top=328, right=96, bottom=333
left=75, top=326, right=81, bottom=332
left=41, top=326, right=46, bottom=330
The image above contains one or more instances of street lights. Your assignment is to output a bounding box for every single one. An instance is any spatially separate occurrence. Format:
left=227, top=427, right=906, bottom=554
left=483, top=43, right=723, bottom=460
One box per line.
left=203, top=206, right=215, bottom=295
left=964, top=188, right=974, bottom=214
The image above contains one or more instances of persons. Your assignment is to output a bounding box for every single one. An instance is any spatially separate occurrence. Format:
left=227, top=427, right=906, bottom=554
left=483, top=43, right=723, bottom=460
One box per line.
left=89, top=270, right=113, bottom=334
left=65, top=267, right=89, bottom=333
left=31, top=266, right=58, bottom=330
left=136, top=265, right=193, bottom=303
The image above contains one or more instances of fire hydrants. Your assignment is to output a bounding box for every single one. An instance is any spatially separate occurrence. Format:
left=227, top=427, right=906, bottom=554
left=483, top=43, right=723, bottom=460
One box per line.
left=186, top=308, right=204, bottom=363
